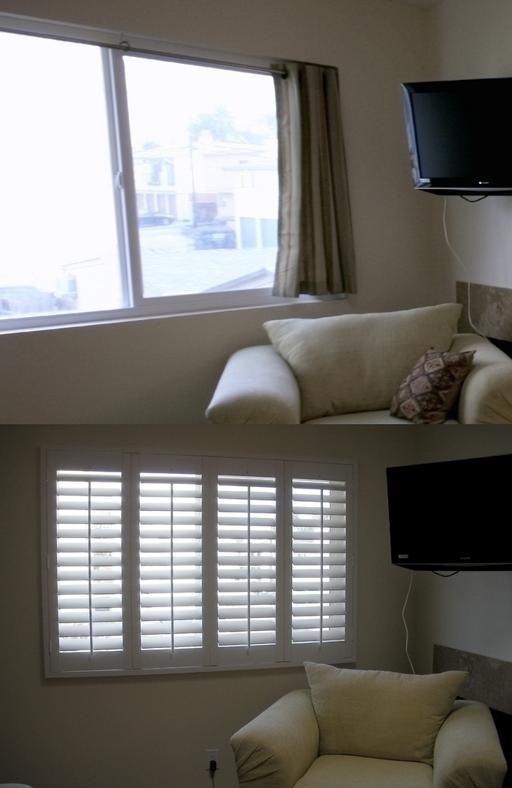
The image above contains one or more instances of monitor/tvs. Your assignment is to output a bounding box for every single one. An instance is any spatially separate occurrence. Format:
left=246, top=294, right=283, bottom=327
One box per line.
left=399, top=76, right=512, bottom=196
left=385, top=453, right=512, bottom=572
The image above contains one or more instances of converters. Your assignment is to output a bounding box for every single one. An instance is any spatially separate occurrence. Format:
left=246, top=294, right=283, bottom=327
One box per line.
left=210, top=760, right=216, bottom=771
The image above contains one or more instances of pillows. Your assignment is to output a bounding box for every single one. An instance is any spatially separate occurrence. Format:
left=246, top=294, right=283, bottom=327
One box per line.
left=302, top=662, right=469, bottom=769
left=264, top=304, right=463, bottom=422
left=390, top=346, right=476, bottom=425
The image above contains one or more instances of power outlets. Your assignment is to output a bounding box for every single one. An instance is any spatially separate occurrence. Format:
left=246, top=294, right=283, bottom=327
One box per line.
left=205, top=749, right=220, bottom=770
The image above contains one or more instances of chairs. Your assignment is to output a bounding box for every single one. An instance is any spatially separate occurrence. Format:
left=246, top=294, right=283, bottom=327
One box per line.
left=229, top=660, right=507, bottom=788
left=205, top=302, right=512, bottom=424
